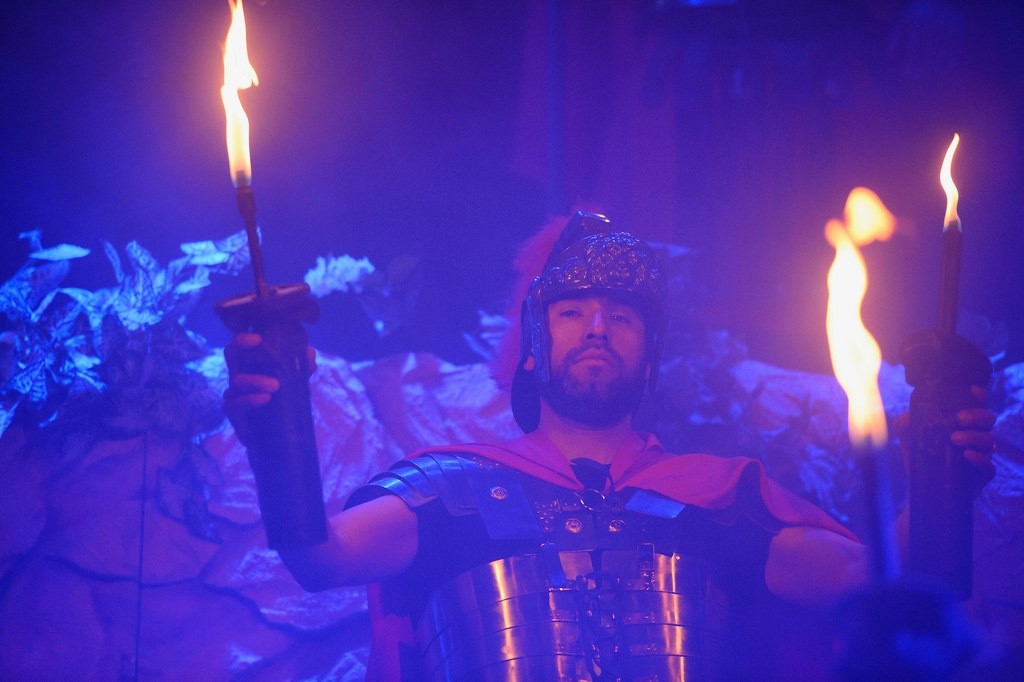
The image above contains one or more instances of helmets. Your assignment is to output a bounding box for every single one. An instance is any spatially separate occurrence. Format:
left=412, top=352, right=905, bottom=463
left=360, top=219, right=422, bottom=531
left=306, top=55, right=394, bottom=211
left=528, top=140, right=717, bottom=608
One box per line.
left=534, top=231, right=676, bottom=316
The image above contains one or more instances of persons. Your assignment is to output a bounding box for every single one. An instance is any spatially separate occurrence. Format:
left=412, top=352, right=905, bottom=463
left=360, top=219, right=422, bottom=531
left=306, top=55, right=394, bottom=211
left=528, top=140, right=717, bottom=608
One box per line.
left=218, top=205, right=995, bottom=682
